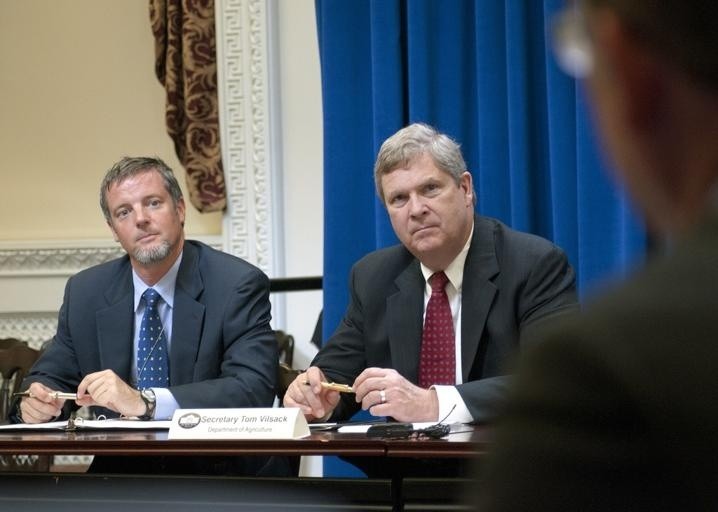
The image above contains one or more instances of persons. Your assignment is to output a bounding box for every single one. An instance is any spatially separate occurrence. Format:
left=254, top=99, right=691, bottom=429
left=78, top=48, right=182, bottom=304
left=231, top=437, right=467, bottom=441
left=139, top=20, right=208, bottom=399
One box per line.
left=461, top=1, right=718, bottom=512
left=6, top=154, right=279, bottom=477
left=283, top=120, right=585, bottom=480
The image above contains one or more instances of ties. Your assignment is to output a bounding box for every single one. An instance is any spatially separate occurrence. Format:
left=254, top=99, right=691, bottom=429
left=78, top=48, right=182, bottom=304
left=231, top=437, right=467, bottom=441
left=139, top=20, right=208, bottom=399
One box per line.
left=136, top=288, right=170, bottom=391
left=415, top=269, right=458, bottom=389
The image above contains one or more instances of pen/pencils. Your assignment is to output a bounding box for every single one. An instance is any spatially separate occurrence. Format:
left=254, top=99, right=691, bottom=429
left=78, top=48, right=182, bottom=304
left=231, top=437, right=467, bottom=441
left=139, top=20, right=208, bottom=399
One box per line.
left=12, top=392, right=77, bottom=400
left=302, top=380, right=354, bottom=393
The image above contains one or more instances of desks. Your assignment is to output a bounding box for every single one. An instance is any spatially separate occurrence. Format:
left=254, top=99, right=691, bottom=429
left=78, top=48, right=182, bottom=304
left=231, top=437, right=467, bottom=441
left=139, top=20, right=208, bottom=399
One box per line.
left=1, top=417, right=492, bottom=512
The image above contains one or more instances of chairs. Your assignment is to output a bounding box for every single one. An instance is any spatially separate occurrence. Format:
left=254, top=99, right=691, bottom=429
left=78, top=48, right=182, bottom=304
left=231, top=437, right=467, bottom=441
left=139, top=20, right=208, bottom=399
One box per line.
left=1, top=338, right=54, bottom=475
left=252, top=330, right=294, bottom=473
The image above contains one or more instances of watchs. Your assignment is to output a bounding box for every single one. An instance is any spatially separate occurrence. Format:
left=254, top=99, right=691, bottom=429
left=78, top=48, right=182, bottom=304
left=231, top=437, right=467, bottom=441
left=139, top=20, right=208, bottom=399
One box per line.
left=137, top=388, right=156, bottom=420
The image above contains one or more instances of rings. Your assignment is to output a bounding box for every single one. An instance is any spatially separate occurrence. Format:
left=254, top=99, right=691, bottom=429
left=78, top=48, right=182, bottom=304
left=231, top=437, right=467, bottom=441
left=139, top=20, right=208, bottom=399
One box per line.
left=379, top=391, right=386, bottom=404
